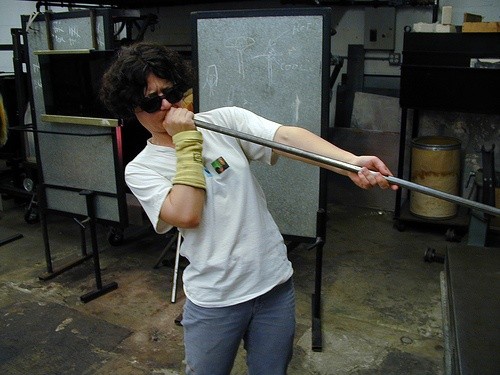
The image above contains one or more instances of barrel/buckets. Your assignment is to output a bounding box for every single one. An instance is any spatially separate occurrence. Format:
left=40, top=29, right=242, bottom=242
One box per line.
left=410, top=136, right=462, bottom=219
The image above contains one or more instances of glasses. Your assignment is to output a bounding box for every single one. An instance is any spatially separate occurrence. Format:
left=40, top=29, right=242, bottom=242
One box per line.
left=134, top=82, right=184, bottom=113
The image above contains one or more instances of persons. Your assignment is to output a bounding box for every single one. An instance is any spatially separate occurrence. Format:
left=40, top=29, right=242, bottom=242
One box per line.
left=98, top=41, right=399, bottom=375
